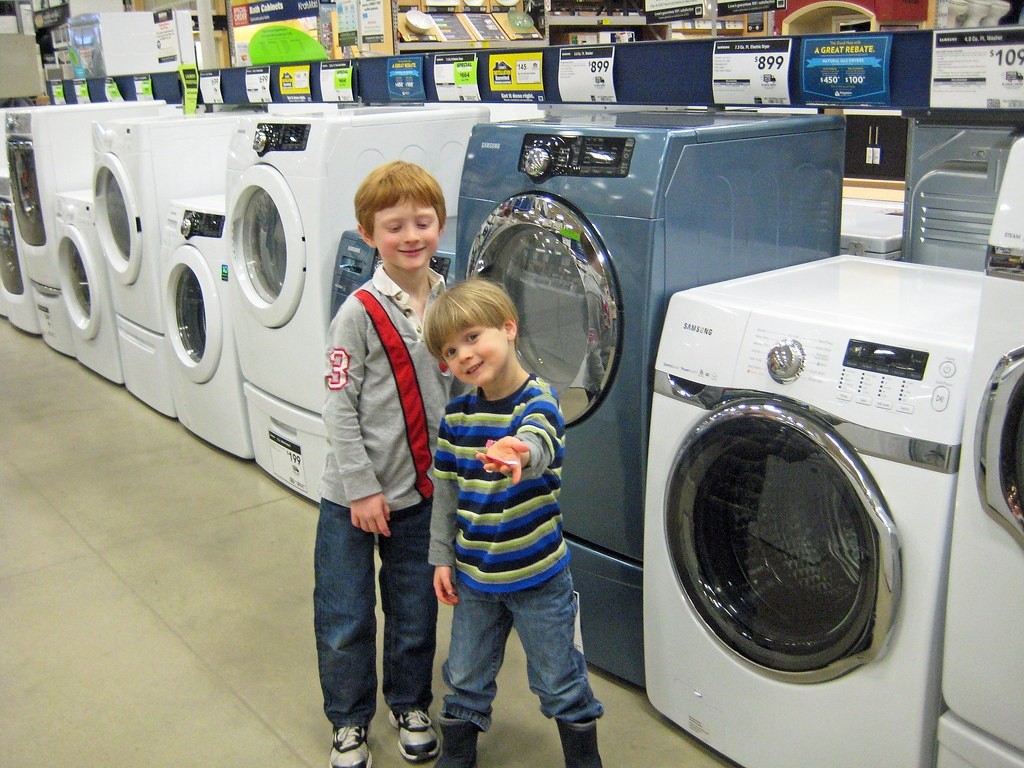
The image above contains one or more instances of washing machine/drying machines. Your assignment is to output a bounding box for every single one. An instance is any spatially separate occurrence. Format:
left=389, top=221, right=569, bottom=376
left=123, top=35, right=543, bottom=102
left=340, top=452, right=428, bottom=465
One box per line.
left=4, top=98, right=1024, bottom=761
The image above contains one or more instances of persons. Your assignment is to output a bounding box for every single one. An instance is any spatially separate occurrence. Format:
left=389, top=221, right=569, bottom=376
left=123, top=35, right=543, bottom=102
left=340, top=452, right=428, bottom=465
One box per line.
left=421, top=278, right=604, bottom=768
left=312, top=159, right=472, bottom=768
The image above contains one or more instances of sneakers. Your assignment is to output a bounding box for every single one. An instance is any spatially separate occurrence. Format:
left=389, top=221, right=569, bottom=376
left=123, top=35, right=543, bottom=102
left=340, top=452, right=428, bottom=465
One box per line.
left=328, top=723, right=372, bottom=768
left=389, top=705, right=441, bottom=762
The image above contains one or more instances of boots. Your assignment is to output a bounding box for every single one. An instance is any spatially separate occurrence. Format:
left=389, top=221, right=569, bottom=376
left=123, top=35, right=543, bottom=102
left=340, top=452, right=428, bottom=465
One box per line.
left=555, top=718, right=602, bottom=768
left=430, top=711, right=478, bottom=768
